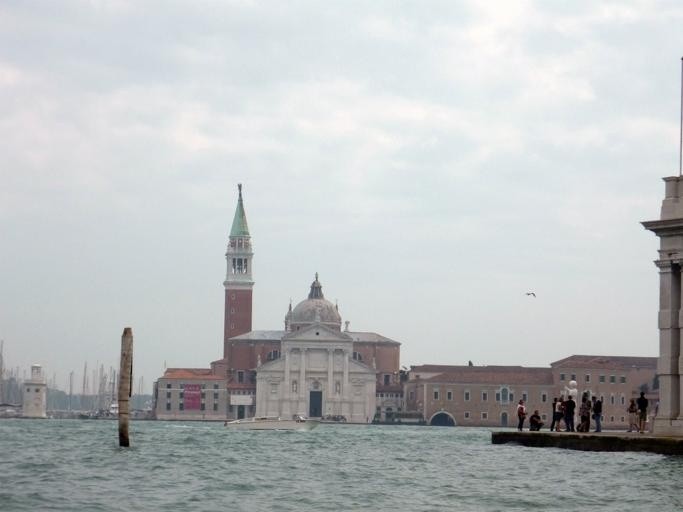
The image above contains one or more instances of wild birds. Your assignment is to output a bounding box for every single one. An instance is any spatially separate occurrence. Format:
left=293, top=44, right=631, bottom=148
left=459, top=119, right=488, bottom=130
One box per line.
left=468, top=361, right=473, bottom=367
left=523, top=292, right=537, bottom=298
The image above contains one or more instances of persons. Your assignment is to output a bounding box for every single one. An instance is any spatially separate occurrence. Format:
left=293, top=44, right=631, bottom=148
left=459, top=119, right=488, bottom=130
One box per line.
left=564, top=379, right=578, bottom=432
left=516, top=399, right=528, bottom=432
left=550, top=392, right=603, bottom=433
left=636, top=391, right=648, bottom=433
left=529, top=409, right=546, bottom=431
left=626, top=399, right=639, bottom=433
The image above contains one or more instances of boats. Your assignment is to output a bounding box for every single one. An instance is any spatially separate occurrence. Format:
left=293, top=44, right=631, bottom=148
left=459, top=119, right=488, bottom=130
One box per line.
left=224, top=417, right=321, bottom=431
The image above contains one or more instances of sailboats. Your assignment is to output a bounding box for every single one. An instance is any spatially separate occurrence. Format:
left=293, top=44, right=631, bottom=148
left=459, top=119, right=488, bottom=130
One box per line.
left=0, top=362, right=167, bottom=420
